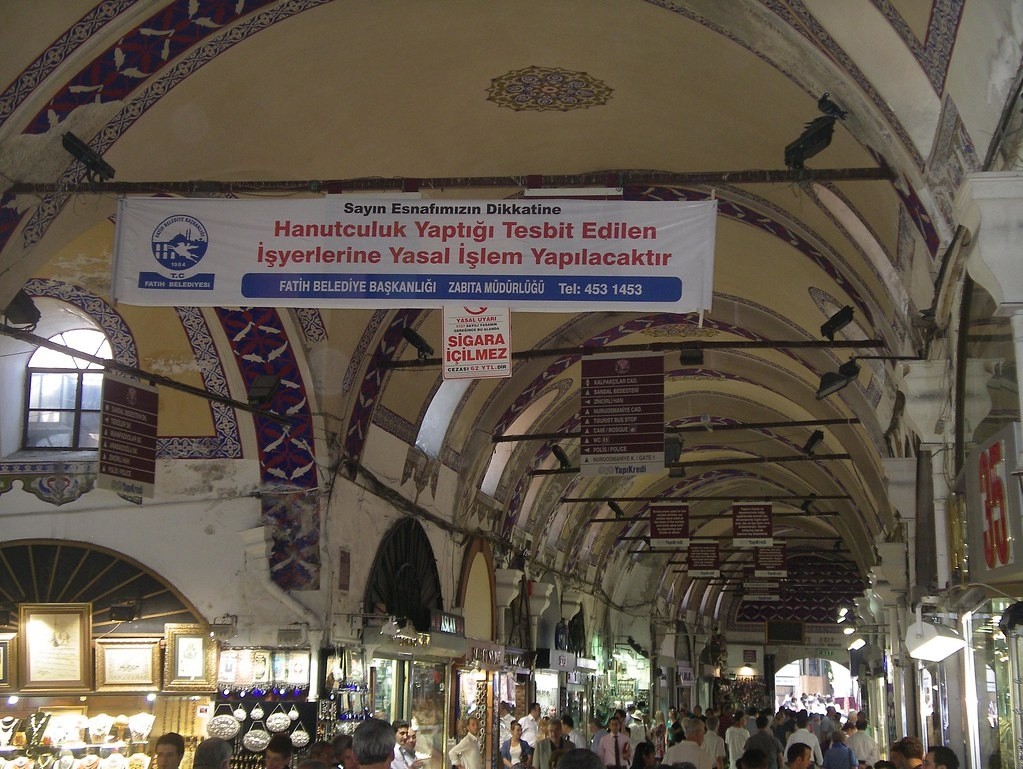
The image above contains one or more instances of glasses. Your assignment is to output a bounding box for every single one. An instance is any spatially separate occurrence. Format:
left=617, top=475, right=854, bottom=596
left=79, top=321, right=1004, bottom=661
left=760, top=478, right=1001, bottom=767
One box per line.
left=923, top=760, right=936, bottom=767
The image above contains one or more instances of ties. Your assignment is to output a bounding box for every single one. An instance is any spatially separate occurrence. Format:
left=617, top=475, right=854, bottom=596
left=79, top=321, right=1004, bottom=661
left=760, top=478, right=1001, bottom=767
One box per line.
left=613, top=735, right=620, bottom=767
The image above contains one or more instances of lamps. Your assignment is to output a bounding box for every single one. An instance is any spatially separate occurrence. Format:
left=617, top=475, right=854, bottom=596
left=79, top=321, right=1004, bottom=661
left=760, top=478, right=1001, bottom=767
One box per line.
left=820, top=305, right=856, bottom=341
left=332, top=612, right=401, bottom=639
left=350, top=616, right=421, bottom=641
left=598, top=634, right=635, bottom=646
left=109, top=596, right=143, bottom=624
left=615, top=643, right=642, bottom=655
left=904, top=603, right=966, bottom=662
left=401, top=325, right=704, bottom=551
left=835, top=604, right=890, bottom=635
left=801, top=430, right=845, bottom=549
left=814, top=354, right=921, bottom=401
left=783, top=115, right=837, bottom=171
left=5, top=291, right=42, bottom=327
left=60, top=130, right=116, bottom=182
left=621, top=648, right=650, bottom=659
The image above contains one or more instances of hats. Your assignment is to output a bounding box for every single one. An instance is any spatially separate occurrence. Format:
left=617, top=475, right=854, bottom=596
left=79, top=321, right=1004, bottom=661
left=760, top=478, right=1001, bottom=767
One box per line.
left=630, top=709, right=642, bottom=721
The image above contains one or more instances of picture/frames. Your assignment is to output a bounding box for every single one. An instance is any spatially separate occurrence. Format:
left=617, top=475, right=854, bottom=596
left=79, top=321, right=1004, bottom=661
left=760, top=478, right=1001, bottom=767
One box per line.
left=163, top=623, right=221, bottom=692
left=0, top=632, right=18, bottom=693
left=94, top=638, right=162, bottom=694
left=18, top=603, right=94, bottom=696
left=37, top=706, right=88, bottom=743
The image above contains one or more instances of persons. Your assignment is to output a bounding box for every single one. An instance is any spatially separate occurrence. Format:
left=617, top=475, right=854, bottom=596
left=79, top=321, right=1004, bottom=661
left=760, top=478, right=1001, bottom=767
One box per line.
left=405, top=725, right=431, bottom=760
left=353, top=719, right=396, bottom=769
left=532, top=718, right=576, bottom=769
left=265, top=735, right=292, bottom=769
left=590, top=718, right=608, bottom=754
left=193, top=738, right=232, bottom=769
left=627, top=692, right=959, bottom=769
left=332, top=734, right=353, bottom=769
left=390, top=719, right=423, bottom=769
left=598, top=717, right=632, bottom=769
left=309, top=741, right=335, bottom=769
left=560, top=715, right=587, bottom=749
left=615, top=710, right=631, bottom=737
left=156, top=732, right=184, bottom=769
left=557, top=748, right=607, bottom=769
left=448, top=717, right=482, bottom=769
left=296, top=759, right=328, bottom=769
left=518, top=703, right=542, bottom=749
left=501, top=720, right=532, bottom=769
left=499, top=701, right=515, bottom=751
